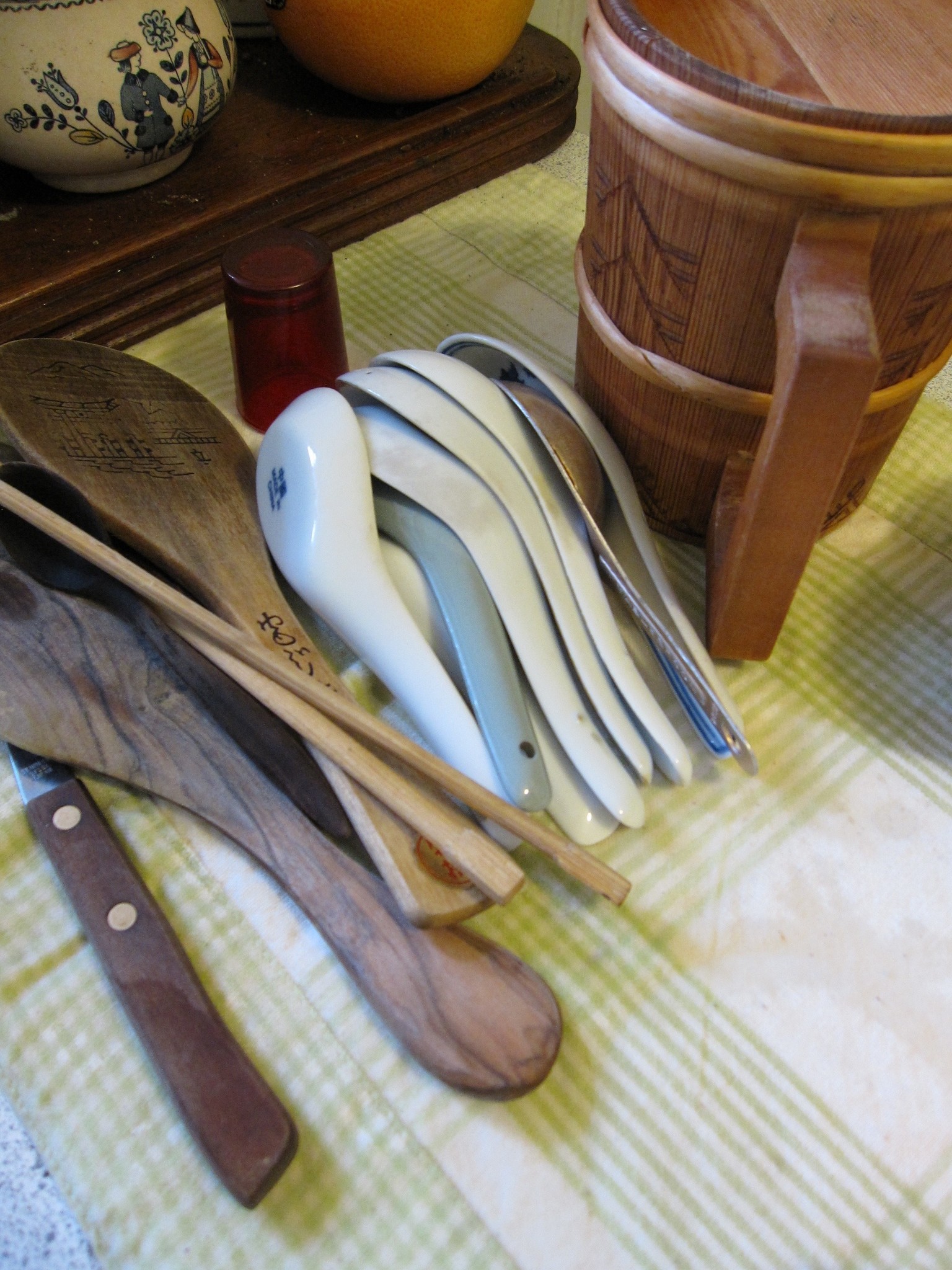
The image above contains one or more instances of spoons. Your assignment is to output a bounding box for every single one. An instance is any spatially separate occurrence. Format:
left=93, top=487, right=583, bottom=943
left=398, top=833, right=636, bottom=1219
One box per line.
left=1, top=461, right=351, bottom=839
left=256, top=331, right=759, bottom=854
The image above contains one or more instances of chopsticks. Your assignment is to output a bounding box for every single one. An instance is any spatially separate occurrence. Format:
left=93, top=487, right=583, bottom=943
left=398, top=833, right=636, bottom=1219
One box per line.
left=1, top=474, right=633, bottom=909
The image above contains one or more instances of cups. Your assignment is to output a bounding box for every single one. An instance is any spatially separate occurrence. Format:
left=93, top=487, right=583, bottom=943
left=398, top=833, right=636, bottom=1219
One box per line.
left=573, top=0, right=952, bottom=661
left=263, top=0, right=534, bottom=103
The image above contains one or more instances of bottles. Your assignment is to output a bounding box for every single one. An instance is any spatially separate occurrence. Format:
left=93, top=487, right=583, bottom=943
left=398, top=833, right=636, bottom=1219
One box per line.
left=0, top=0, right=238, bottom=193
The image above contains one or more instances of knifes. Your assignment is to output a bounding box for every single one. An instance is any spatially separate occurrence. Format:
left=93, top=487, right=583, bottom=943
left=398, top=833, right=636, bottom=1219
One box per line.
left=6, top=744, right=294, bottom=1207
